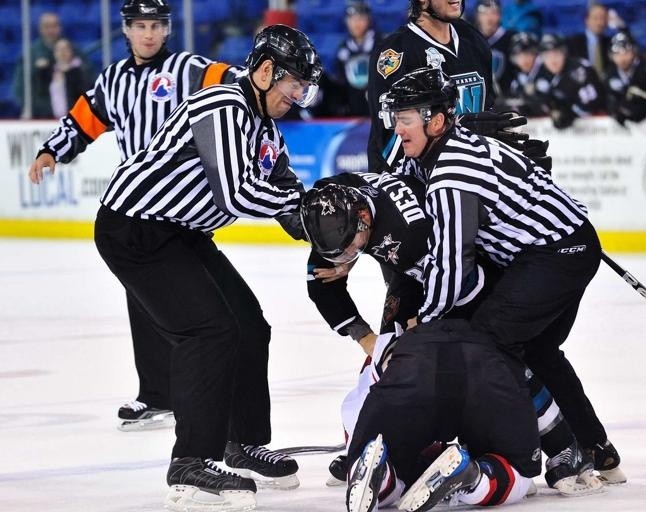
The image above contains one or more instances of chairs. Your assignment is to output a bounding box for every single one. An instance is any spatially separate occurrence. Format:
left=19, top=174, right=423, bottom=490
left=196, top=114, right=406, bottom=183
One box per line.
left=1, top=0, right=646, bottom=121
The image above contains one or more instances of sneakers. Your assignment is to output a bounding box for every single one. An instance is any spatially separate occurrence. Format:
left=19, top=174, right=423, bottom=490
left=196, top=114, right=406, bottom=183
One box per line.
left=544, top=446, right=588, bottom=488
left=165, top=456, right=257, bottom=493
left=224, top=440, right=299, bottom=478
left=344, top=439, right=388, bottom=512
left=591, top=434, right=621, bottom=470
left=118, top=396, right=173, bottom=419
left=403, top=449, right=481, bottom=512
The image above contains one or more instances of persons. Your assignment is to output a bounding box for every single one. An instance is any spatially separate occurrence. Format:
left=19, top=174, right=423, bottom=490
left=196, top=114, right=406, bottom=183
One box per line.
left=382, top=65, right=626, bottom=471
left=362, top=1, right=529, bottom=173
left=302, top=167, right=596, bottom=487
left=27, top=2, right=248, bottom=422
left=343, top=316, right=543, bottom=511
left=92, top=24, right=359, bottom=498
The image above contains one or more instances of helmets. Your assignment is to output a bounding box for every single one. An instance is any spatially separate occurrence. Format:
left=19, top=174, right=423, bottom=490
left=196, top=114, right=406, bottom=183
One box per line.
left=607, top=31, right=638, bottom=54
left=379, top=68, right=460, bottom=132
left=510, top=32, right=570, bottom=56
left=119, top=0, right=171, bottom=40
left=246, top=24, right=323, bottom=109
left=299, top=182, right=369, bottom=262
